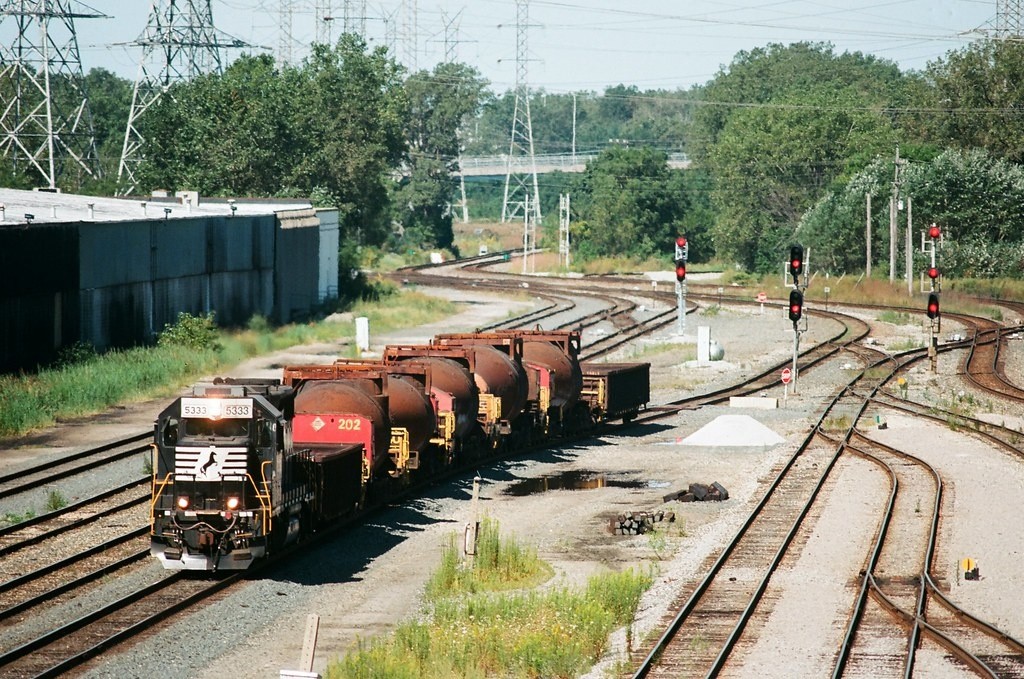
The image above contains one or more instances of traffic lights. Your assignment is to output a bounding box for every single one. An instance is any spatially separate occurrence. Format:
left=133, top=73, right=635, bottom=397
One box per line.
left=927, top=269, right=939, bottom=278
left=676, top=259, right=685, bottom=282
left=677, top=237, right=685, bottom=247
left=789, top=289, right=802, bottom=321
left=927, top=291, right=939, bottom=319
left=928, top=227, right=940, bottom=238
left=790, top=243, right=803, bottom=276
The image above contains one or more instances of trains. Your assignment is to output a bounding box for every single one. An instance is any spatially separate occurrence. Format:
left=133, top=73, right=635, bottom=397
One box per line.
left=150, top=326, right=651, bottom=578
